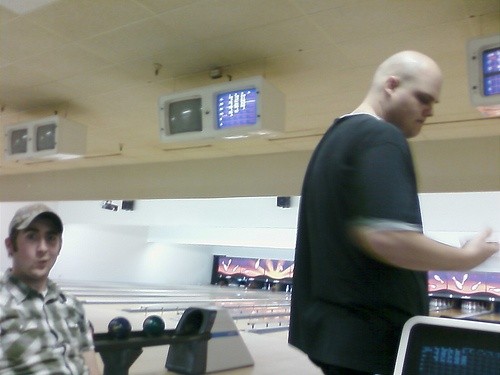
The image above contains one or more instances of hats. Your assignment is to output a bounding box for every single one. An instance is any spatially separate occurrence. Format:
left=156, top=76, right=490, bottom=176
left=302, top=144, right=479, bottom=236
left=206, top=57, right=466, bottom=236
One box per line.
left=7, top=202, right=63, bottom=238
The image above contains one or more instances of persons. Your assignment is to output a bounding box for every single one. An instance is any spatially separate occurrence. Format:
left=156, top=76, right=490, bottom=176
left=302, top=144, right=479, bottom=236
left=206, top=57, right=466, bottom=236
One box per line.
left=288, top=50, right=500, bottom=375
left=1, top=204, right=100, bottom=375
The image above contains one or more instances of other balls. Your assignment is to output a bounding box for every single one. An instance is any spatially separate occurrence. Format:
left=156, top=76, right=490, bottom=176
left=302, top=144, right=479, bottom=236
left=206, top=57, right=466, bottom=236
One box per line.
left=144, top=315, right=167, bottom=336
left=107, top=317, right=132, bottom=340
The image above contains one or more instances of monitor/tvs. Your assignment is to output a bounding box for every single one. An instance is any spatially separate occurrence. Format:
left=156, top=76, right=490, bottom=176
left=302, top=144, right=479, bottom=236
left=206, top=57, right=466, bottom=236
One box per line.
left=207, top=75, right=285, bottom=139
left=31, top=114, right=88, bottom=161
left=4, top=122, right=32, bottom=161
left=157, top=84, right=208, bottom=145
left=393, top=315, right=500, bottom=375
left=466, top=32, right=500, bottom=117
